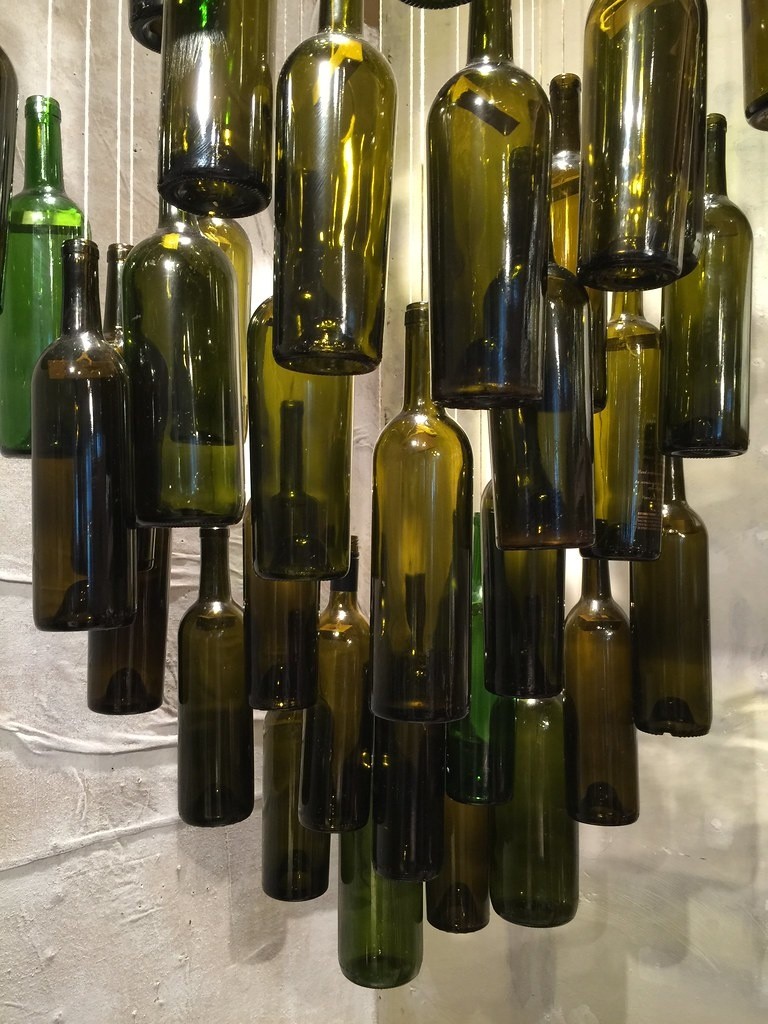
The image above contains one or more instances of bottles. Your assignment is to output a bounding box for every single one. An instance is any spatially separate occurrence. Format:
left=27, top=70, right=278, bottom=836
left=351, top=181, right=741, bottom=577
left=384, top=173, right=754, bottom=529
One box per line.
left=565, top=520, right=639, bottom=826
left=262, top=705, right=332, bottom=902
left=319, top=537, right=373, bottom=833
left=427, top=800, right=488, bottom=933
left=247, top=170, right=353, bottom=582
left=338, top=774, right=424, bottom=990
left=482, top=407, right=565, bottom=700
left=427, top=1, right=553, bottom=410
left=371, top=302, right=473, bottom=726
left=438, top=512, right=517, bottom=805
left=372, top=572, right=446, bottom=878
left=660, top=112, right=753, bottom=456
left=273, top=0, right=398, bottom=377
left=582, top=290, right=664, bottom=561
left=241, top=498, right=319, bottom=712
left=487, top=696, right=579, bottom=927
left=178, top=527, right=253, bottom=827
left=631, top=452, right=711, bottom=736
left=124, top=196, right=252, bottom=527
left=30, top=239, right=168, bottom=716
left=742, top=0, right=767, bottom=131
left=546, top=73, right=609, bottom=416
left=1, top=0, right=219, bottom=457
left=579, top=0, right=707, bottom=291
left=488, top=146, right=582, bottom=409
